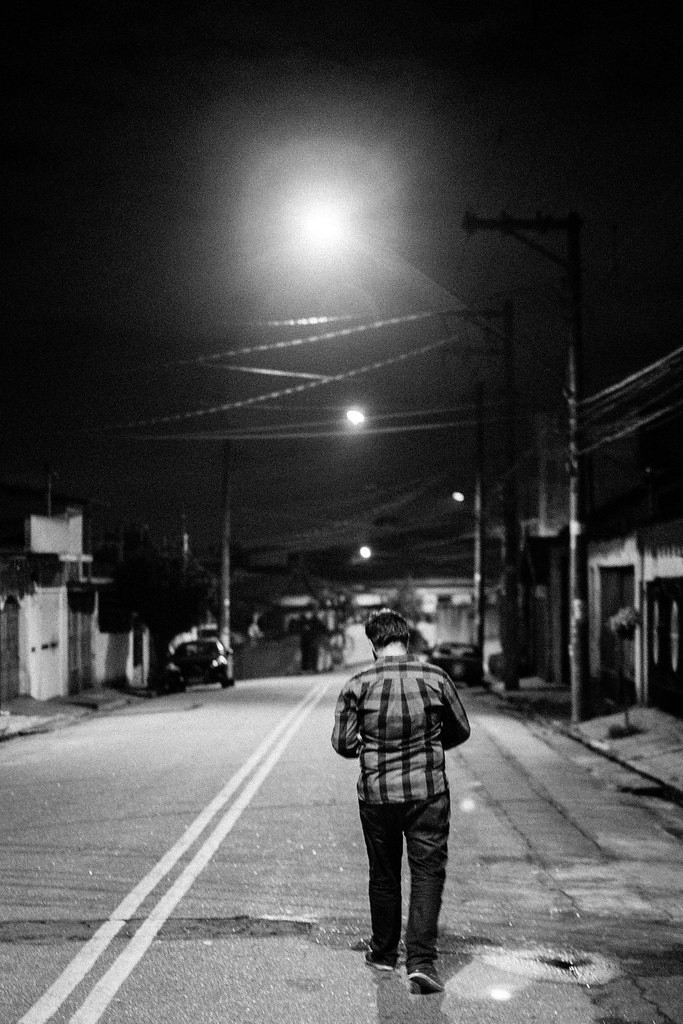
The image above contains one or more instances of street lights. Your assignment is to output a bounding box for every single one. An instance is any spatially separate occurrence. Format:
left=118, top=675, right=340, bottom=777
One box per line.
left=293, top=192, right=590, bottom=724
left=342, top=408, right=485, bottom=693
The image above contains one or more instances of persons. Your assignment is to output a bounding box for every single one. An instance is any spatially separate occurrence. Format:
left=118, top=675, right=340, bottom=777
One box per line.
left=331, top=608, right=470, bottom=993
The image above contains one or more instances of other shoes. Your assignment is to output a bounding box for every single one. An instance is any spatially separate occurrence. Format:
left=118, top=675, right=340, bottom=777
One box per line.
left=363, top=950, right=397, bottom=971
left=407, top=961, right=444, bottom=993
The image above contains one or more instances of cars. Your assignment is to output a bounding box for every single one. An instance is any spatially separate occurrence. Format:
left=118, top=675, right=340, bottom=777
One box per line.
left=428, top=640, right=476, bottom=688
left=165, top=632, right=236, bottom=690
